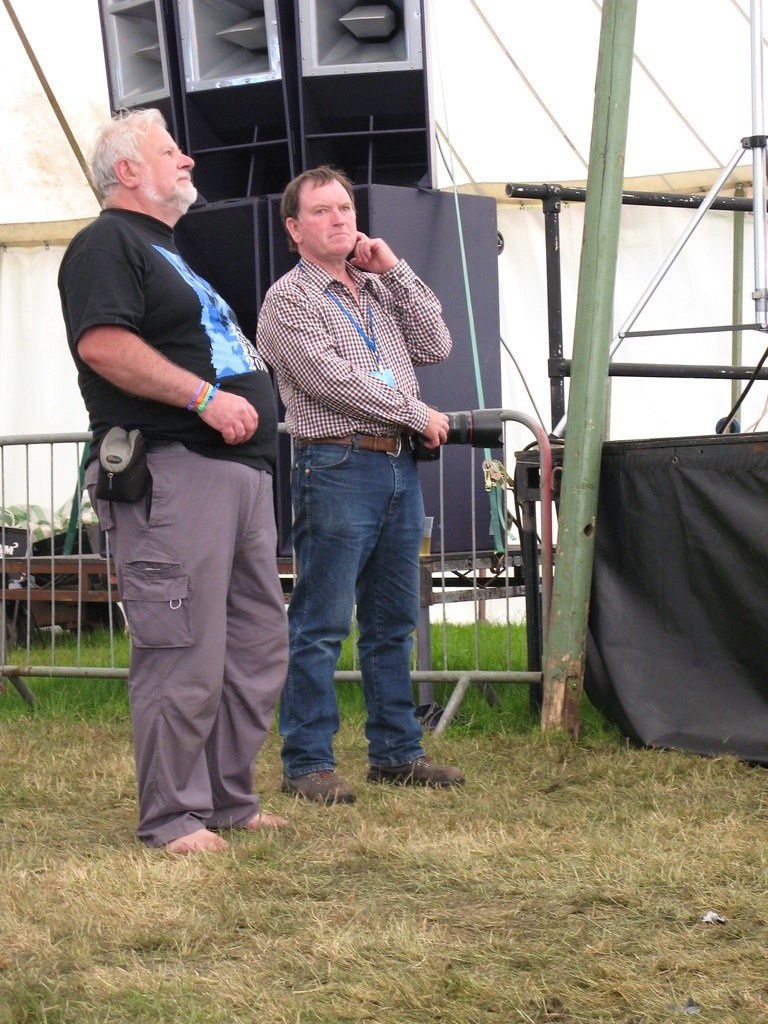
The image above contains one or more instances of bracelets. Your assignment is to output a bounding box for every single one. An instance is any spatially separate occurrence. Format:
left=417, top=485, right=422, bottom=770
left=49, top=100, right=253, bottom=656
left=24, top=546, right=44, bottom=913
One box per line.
left=188, top=380, right=220, bottom=413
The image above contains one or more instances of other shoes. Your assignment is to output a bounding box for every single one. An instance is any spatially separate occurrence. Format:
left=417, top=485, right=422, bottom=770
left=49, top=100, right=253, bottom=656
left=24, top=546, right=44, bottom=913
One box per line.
left=367, top=755, right=466, bottom=790
left=281, top=769, right=357, bottom=807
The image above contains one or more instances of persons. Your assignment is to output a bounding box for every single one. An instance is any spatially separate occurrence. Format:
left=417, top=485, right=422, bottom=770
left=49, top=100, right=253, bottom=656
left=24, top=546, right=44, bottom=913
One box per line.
left=59, top=109, right=288, bottom=852
left=256, top=167, right=465, bottom=803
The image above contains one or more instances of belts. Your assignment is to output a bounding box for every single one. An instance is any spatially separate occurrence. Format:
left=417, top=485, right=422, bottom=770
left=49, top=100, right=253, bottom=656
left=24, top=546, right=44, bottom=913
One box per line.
left=303, top=432, right=414, bottom=457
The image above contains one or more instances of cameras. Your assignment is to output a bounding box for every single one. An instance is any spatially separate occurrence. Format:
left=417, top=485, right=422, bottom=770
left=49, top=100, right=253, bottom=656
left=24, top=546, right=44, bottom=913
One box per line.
left=411, top=408, right=505, bottom=461
